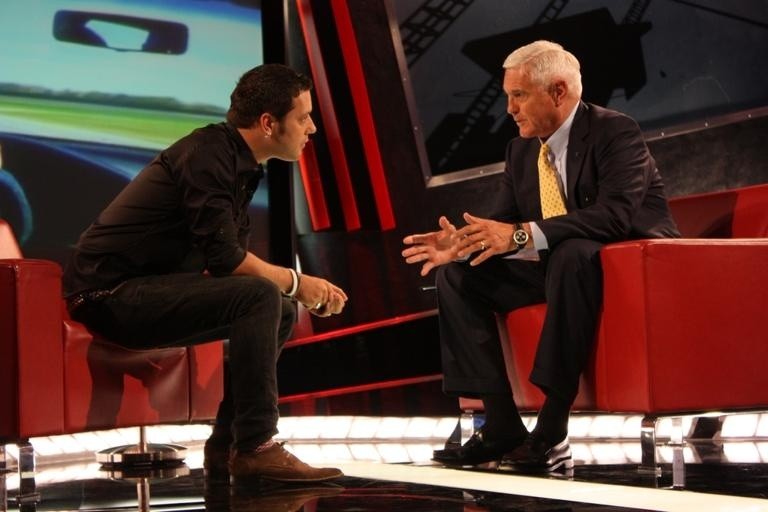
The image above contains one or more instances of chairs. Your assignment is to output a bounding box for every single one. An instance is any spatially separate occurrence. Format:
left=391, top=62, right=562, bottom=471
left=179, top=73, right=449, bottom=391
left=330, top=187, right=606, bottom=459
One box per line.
left=457, top=184, right=768, bottom=478
left=0, top=216, right=224, bottom=512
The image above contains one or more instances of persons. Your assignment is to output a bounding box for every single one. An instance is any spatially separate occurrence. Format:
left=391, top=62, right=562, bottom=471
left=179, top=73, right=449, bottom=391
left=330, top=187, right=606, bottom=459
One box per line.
left=400, top=37, right=682, bottom=478
left=61, top=63, right=347, bottom=485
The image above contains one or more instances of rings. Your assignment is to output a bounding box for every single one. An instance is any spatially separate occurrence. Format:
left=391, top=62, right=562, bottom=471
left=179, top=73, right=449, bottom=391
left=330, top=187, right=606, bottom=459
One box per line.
left=315, top=301, right=321, bottom=310
left=480, top=240, right=485, bottom=251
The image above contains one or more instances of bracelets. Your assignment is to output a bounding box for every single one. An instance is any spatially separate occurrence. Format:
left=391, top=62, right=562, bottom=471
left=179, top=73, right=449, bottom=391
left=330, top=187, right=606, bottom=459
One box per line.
left=280, top=268, right=299, bottom=298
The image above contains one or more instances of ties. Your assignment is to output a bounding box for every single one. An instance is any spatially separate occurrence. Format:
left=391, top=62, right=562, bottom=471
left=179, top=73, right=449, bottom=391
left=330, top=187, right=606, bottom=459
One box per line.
left=537, top=143, right=568, bottom=219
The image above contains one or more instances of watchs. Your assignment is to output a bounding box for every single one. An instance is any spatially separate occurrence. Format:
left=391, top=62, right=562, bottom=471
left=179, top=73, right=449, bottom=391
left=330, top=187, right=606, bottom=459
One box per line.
left=512, top=222, right=529, bottom=249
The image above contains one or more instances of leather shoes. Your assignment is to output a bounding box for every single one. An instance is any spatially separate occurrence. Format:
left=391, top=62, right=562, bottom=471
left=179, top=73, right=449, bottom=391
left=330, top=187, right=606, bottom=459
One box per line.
left=433, top=422, right=574, bottom=470
left=204, top=438, right=345, bottom=487
